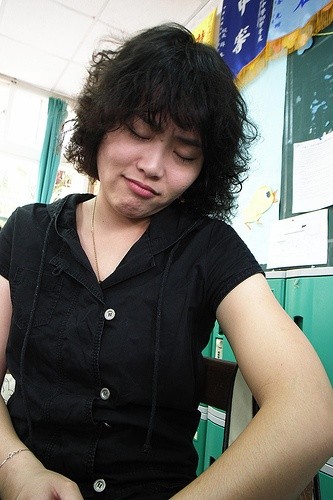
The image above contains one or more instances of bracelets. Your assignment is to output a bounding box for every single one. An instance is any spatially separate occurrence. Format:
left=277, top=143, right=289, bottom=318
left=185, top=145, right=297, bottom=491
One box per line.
left=0, top=448, right=30, bottom=468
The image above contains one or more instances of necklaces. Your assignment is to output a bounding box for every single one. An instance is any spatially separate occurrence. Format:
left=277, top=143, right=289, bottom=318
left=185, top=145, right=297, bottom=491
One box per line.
left=91, top=196, right=100, bottom=284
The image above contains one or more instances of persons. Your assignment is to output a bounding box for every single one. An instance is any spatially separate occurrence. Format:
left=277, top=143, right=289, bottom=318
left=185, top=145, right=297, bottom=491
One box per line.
left=1, top=23, right=333, bottom=500
